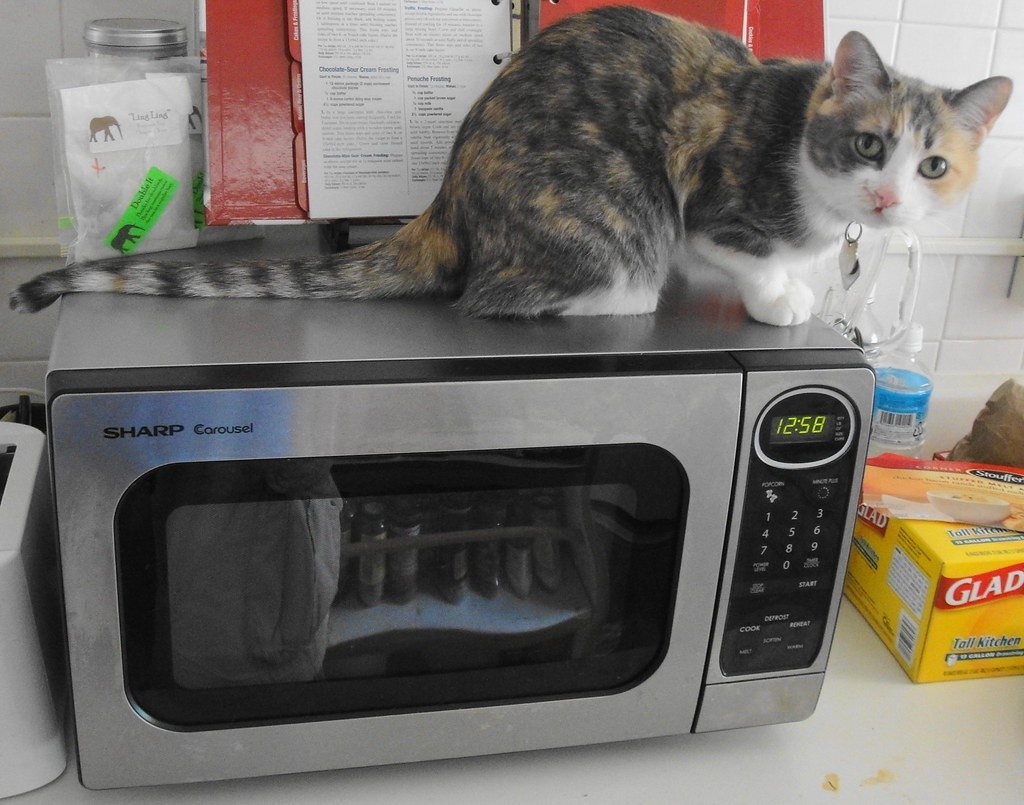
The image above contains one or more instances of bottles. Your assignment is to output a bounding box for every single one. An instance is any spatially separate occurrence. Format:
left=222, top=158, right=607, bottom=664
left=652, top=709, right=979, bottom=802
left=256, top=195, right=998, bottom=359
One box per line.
left=866, top=323, right=935, bottom=464
left=79, top=16, right=190, bottom=59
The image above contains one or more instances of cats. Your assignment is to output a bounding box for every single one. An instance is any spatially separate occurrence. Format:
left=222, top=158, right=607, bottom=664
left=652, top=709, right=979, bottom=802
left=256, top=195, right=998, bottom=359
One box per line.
left=6, top=5, right=1012, bottom=327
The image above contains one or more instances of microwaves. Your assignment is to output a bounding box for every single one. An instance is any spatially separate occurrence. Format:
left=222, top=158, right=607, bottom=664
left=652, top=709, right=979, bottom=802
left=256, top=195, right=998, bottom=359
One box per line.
left=43, top=277, right=877, bottom=794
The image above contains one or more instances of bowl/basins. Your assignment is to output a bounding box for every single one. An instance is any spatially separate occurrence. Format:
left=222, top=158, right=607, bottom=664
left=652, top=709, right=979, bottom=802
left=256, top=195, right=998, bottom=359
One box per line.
left=926, top=490, right=1011, bottom=525
